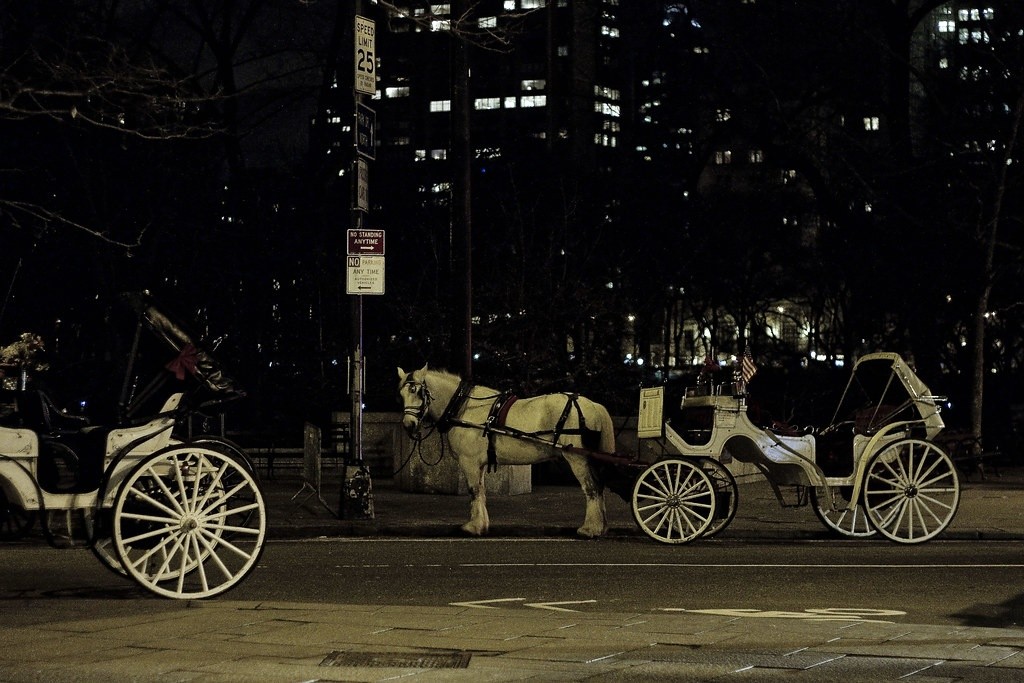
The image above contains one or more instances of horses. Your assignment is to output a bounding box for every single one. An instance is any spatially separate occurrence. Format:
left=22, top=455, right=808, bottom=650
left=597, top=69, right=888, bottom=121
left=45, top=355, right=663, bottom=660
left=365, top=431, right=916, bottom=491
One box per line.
left=394, top=361, right=616, bottom=537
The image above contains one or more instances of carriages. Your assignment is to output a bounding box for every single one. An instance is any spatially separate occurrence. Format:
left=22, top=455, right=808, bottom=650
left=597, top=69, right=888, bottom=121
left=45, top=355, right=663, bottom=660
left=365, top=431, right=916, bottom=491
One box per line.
left=398, top=337, right=963, bottom=548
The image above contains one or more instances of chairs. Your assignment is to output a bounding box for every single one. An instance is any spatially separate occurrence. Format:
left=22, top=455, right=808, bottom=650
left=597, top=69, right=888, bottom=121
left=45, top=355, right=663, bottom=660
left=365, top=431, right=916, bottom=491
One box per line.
left=831, top=396, right=914, bottom=463
left=73, top=390, right=189, bottom=488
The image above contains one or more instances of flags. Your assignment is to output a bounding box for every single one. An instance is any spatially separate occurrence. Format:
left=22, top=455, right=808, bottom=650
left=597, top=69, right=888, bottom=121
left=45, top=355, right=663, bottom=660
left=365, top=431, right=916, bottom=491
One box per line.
left=740, top=346, right=757, bottom=384
